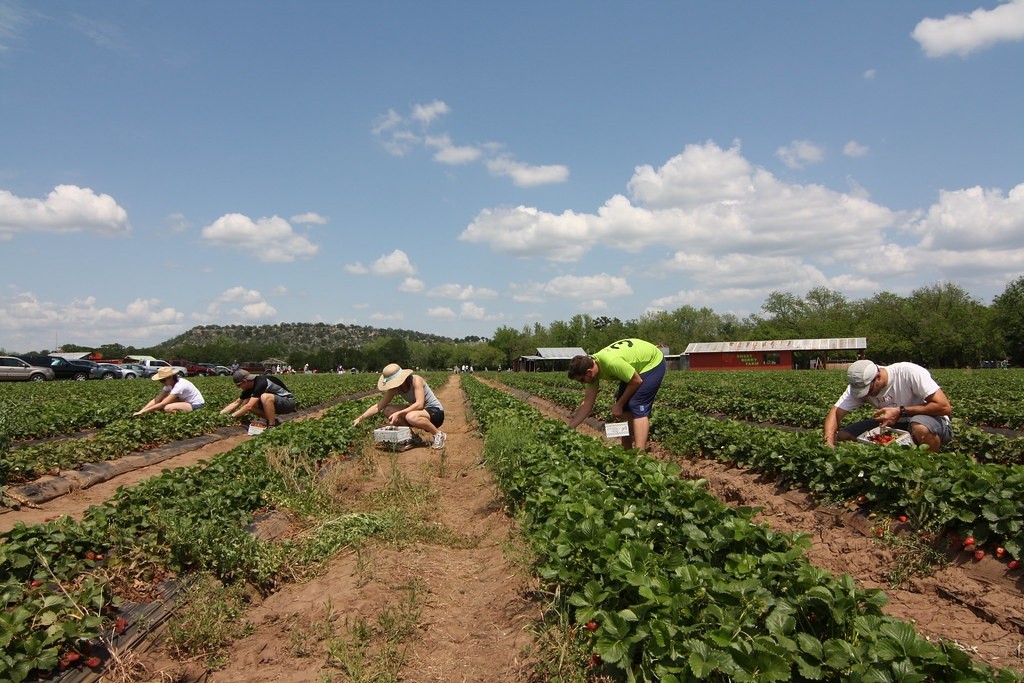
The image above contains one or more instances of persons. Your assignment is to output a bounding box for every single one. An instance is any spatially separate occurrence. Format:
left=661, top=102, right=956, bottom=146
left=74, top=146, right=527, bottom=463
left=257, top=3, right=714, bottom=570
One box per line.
left=453, top=364, right=476, bottom=375
left=823, top=358, right=954, bottom=454
left=133, top=366, right=206, bottom=417
left=219, top=368, right=296, bottom=430
left=337, top=365, right=344, bottom=374
left=352, top=363, right=447, bottom=452
left=303, top=363, right=309, bottom=371
left=566, top=338, right=667, bottom=456
left=264, top=365, right=297, bottom=375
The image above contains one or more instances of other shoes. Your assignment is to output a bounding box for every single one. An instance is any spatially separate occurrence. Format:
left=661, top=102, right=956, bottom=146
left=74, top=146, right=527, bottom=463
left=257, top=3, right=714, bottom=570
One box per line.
left=267, top=418, right=280, bottom=426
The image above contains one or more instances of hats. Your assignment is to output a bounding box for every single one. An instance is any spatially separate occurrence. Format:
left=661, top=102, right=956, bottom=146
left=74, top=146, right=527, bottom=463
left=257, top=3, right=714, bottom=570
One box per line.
left=847, top=359, right=878, bottom=399
left=232, top=369, right=259, bottom=383
left=377, top=363, right=413, bottom=391
left=151, top=367, right=180, bottom=381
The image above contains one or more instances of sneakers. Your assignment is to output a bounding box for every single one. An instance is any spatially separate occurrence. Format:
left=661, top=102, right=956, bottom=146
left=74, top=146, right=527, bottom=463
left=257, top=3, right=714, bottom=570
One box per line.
left=431, top=430, right=447, bottom=450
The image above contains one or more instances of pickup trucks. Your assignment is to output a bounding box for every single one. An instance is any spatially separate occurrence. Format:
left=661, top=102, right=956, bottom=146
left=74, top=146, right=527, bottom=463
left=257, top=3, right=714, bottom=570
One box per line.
left=122, top=359, right=188, bottom=379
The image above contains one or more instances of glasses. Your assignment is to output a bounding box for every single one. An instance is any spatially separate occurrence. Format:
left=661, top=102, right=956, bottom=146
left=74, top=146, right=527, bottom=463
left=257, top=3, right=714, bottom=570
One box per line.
left=578, top=369, right=588, bottom=383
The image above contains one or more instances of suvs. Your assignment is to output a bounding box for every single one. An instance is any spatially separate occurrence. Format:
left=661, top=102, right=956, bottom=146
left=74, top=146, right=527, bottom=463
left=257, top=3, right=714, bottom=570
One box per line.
left=239, top=362, right=273, bottom=376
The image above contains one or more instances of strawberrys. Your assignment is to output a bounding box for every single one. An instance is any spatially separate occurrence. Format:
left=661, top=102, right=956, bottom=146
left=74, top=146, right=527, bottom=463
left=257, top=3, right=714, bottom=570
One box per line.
left=866, top=431, right=900, bottom=446
left=875, top=516, right=1022, bottom=570
left=33, top=551, right=126, bottom=667
left=586, top=620, right=598, bottom=630
left=589, top=652, right=602, bottom=665
left=250, top=422, right=266, bottom=428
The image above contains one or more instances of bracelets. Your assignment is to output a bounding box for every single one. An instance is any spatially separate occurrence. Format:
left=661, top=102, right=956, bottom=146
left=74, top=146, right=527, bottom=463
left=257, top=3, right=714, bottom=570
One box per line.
left=900, top=406, right=906, bottom=417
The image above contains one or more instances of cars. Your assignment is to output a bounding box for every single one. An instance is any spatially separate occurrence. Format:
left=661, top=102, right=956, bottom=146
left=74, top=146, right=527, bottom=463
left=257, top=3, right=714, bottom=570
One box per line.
left=0, top=356, right=55, bottom=383
left=32, top=356, right=97, bottom=382
left=117, top=364, right=146, bottom=372
left=217, top=366, right=233, bottom=374
left=167, top=360, right=217, bottom=377
left=68, top=359, right=138, bottom=381
left=197, top=363, right=230, bottom=377
